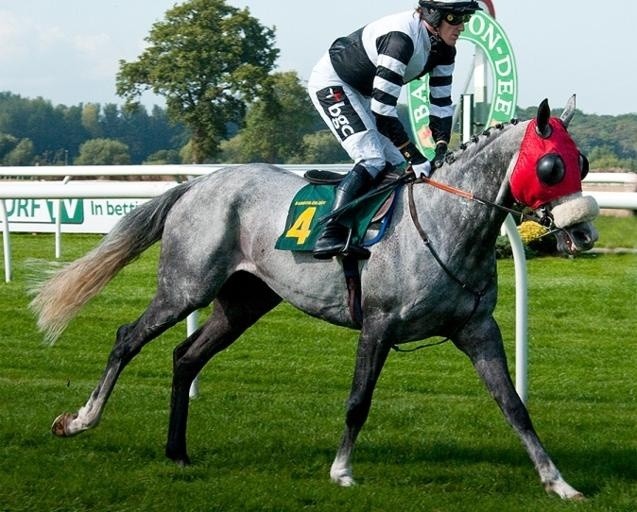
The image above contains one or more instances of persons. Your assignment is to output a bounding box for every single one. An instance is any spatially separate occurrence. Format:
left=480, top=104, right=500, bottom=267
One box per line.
left=307, top=0, right=476, bottom=258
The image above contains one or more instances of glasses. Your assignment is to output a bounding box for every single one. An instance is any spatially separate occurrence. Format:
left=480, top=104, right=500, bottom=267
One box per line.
left=441, top=13, right=469, bottom=25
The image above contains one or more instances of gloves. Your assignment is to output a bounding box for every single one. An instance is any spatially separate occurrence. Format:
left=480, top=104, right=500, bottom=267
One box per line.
left=398, top=140, right=447, bottom=179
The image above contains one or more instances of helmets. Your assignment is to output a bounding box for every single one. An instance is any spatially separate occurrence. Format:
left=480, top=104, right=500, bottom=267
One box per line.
left=419, top=0, right=483, bottom=12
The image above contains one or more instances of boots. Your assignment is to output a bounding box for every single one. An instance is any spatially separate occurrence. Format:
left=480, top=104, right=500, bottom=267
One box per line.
left=313, top=164, right=374, bottom=260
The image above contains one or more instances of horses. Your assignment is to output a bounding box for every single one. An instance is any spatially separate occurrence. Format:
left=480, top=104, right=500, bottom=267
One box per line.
left=19, top=92, right=600, bottom=502
